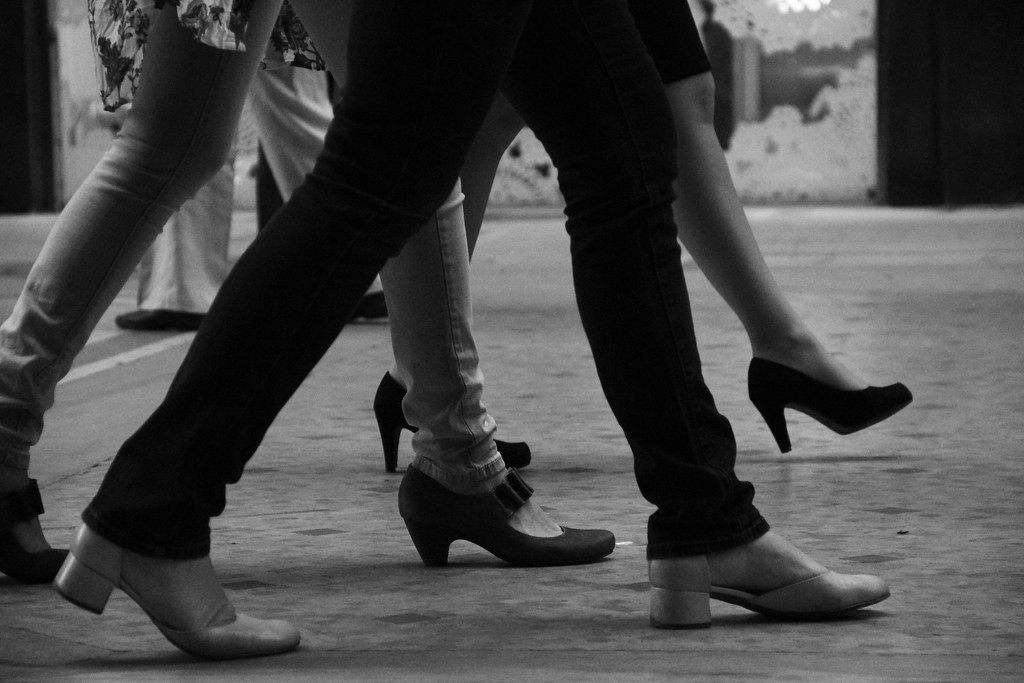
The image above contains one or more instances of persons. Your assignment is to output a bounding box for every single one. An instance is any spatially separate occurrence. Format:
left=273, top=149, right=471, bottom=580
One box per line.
left=86, top=0, right=388, bottom=332
left=52, top=0, right=892, bottom=661
left=0, top=0, right=616, bottom=582
left=374, top=0, right=913, bottom=473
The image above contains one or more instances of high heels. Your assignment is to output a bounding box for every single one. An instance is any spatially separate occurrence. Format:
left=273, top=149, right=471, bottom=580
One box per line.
left=372, top=370, right=532, bottom=472
left=397, top=464, right=615, bottom=567
left=54, top=522, right=301, bottom=658
left=647, top=554, right=891, bottom=628
left=0, top=477, right=71, bottom=583
left=747, top=357, right=913, bottom=454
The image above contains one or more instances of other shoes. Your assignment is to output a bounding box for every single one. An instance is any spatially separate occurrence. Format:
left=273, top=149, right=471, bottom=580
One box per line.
left=349, top=291, right=390, bottom=324
left=114, top=306, right=203, bottom=331
